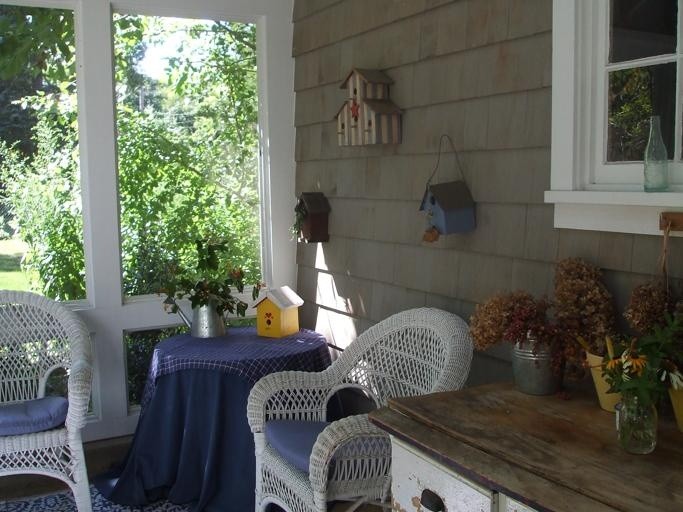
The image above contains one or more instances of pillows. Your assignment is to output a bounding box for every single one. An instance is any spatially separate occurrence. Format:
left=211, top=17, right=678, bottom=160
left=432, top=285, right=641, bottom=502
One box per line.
left=264, top=420, right=359, bottom=475
left=0, top=397, right=68, bottom=436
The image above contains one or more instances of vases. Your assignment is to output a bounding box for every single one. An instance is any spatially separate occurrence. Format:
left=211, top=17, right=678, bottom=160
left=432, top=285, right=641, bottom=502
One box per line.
left=665, top=384, right=683, bottom=431
left=168, top=296, right=230, bottom=338
left=587, top=351, right=626, bottom=412
left=616, top=393, right=659, bottom=456
left=513, top=331, right=565, bottom=396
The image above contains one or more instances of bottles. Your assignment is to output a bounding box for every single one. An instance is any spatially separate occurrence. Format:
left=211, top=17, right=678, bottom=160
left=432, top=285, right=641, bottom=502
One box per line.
left=644, top=114, right=669, bottom=192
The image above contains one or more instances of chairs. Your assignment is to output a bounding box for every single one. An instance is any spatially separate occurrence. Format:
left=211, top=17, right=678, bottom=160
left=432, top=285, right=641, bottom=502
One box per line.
left=0, top=290, right=105, bottom=512
left=246, top=308, right=474, bottom=512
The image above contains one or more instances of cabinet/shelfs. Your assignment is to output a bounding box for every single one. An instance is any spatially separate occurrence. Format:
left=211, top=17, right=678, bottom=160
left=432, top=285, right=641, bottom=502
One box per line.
left=369, top=384, right=683, bottom=512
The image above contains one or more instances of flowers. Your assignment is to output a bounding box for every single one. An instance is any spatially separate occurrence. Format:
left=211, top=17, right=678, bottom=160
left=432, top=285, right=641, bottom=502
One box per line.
left=470, top=292, right=565, bottom=378
left=155, top=235, right=268, bottom=317
left=552, top=257, right=618, bottom=356
left=623, top=283, right=683, bottom=365
left=596, top=310, right=683, bottom=405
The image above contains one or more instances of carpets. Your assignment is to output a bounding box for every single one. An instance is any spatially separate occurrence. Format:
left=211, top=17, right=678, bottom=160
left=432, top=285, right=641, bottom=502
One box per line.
left=0, top=481, right=190, bottom=512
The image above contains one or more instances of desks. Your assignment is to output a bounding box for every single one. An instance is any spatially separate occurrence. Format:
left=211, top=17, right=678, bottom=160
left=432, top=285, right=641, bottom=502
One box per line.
left=94, top=328, right=327, bottom=512
